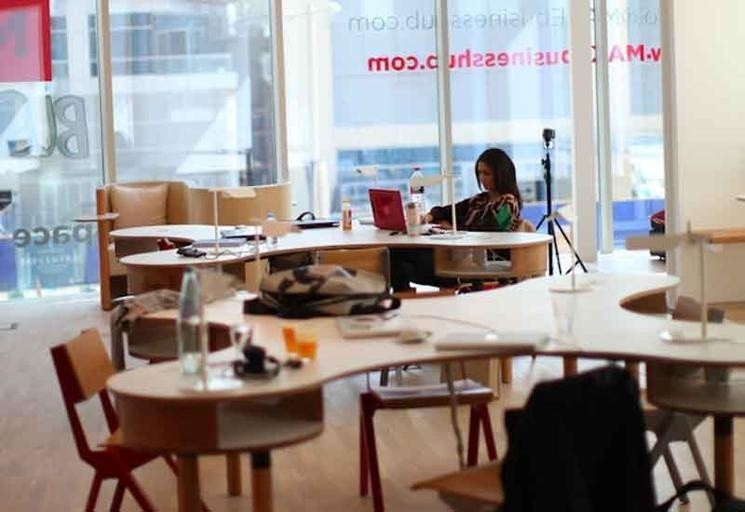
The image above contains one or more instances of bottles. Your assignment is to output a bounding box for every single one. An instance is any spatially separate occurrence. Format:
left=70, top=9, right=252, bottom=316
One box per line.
left=407, top=203, right=419, bottom=237
left=177, top=266, right=206, bottom=371
left=340, top=199, right=352, bottom=230
left=266, top=211, right=279, bottom=245
left=409, top=166, right=427, bottom=213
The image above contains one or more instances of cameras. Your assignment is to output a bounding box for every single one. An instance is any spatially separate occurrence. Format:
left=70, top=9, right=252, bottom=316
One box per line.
left=544, top=129, right=555, bottom=139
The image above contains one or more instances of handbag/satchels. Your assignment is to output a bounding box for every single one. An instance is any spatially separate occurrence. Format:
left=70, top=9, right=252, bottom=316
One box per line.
left=260, top=263, right=385, bottom=318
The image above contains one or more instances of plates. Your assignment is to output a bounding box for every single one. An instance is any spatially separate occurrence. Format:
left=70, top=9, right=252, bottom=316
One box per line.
left=235, top=356, right=280, bottom=378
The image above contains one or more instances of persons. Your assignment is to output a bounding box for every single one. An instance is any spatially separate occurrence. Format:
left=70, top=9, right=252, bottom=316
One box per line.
left=375, top=147, right=524, bottom=295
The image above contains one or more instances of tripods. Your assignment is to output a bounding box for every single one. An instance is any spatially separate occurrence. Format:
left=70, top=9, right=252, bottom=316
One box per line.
left=536, top=143, right=587, bottom=275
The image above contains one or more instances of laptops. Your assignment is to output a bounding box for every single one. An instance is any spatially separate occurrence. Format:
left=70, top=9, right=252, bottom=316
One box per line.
left=220, top=228, right=265, bottom=240
left=369, top=189, right=442, bottom=234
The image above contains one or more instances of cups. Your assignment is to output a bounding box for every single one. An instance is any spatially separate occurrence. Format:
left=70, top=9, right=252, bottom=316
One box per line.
left=232, top=326, right=267, bottom=368
left=276, top=324, right=320, bottom=358
